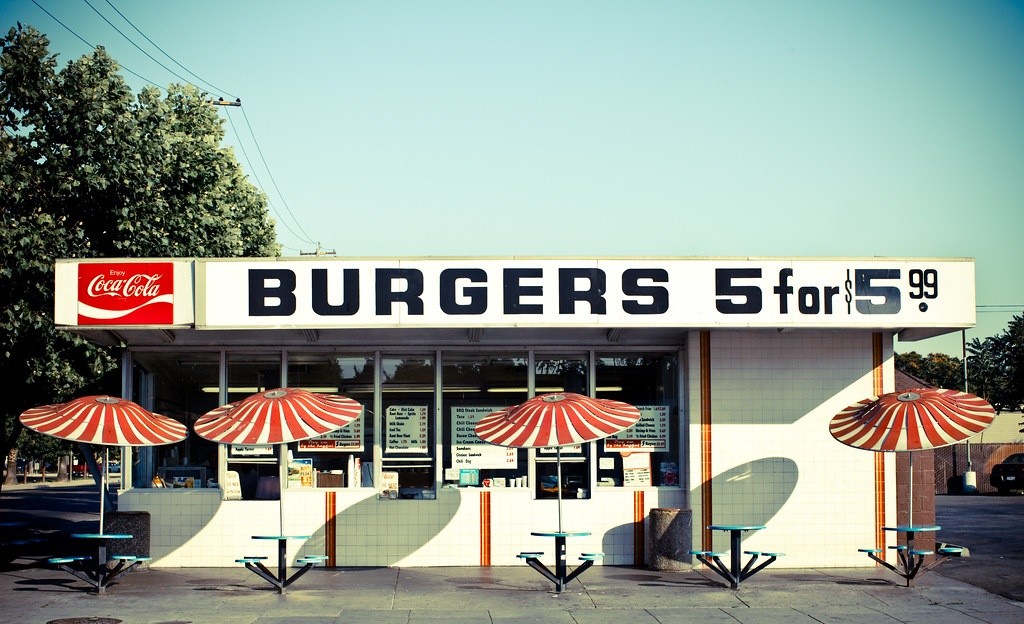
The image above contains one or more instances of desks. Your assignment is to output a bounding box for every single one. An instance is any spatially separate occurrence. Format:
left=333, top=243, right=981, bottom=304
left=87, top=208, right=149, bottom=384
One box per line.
left=71, top=534, right=134, bottom=593
left=882, top=525, right=941, bottom=587
left=531, top=531, right=592, bottom=593
left=708, top=525, right=768, bottom=588
left=251, top=534, right=312, bottom=595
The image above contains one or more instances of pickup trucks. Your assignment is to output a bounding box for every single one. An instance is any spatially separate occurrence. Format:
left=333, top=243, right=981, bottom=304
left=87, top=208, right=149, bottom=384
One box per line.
left=67, top=458, right=102, bottom=477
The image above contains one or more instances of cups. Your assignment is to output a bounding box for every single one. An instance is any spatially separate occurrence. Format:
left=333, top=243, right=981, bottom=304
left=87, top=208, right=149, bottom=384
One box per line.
left=516, top=478, right=522, bottom=487
left=522, top=476, right=527, bottom=487
left=509, top=479, right=516, bottom=487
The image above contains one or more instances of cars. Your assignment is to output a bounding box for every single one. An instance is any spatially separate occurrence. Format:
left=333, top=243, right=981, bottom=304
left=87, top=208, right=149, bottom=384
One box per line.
left=15, top=459, right=30, bottom=474
left=105, top=462, right=120, bottom=473
left=988, top=453, right=1024, bottom=493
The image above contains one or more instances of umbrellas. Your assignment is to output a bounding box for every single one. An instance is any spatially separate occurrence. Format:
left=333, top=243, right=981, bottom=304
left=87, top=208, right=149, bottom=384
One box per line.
left=20, top=395, right=188, bottom=535
left=194, top=387, right=362, bottom=537
left=474, top=392, right=641, bottom=533
left=830, top=387, right=996, bottom=528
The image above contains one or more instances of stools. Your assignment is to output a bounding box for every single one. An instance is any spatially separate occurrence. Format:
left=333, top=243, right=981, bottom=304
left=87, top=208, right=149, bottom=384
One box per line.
left=689, top=551, right=786, bottom=582
left=235, top=556, right=330, bottom=589
left=48, top=555, right=152, bottom=588
left=516, top=552, right=605, bottom=585
left=858, top=545, right=963, bottom=580
